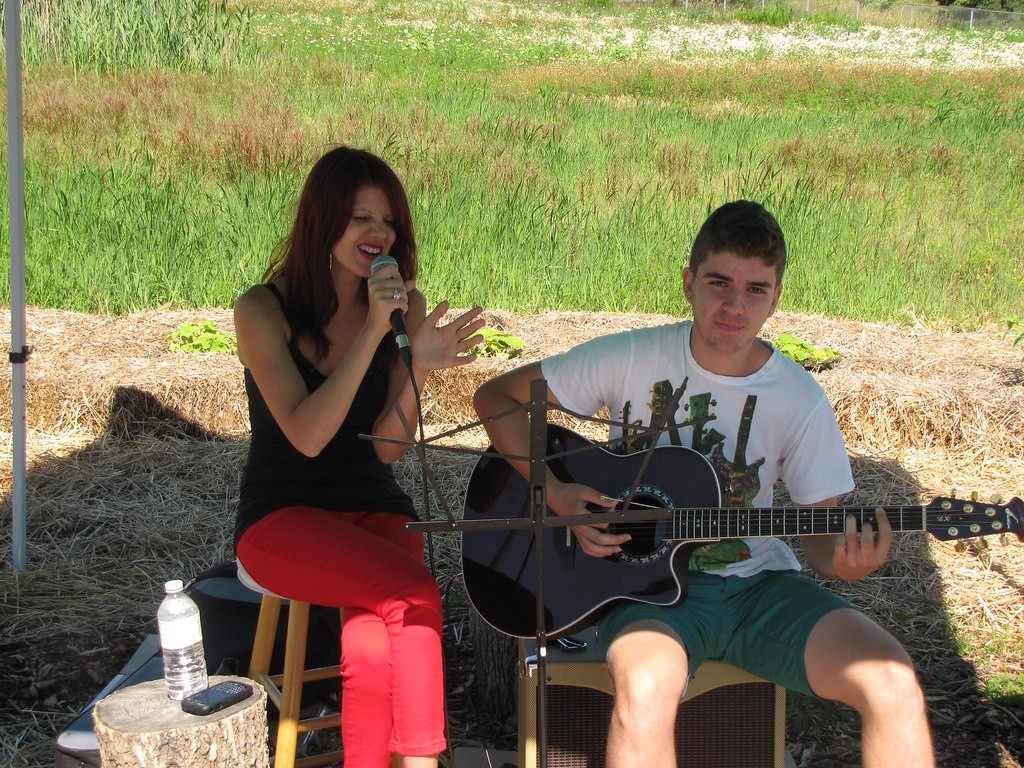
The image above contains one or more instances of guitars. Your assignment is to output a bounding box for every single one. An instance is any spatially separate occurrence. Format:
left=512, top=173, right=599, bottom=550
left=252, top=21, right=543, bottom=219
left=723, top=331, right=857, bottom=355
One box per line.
left=459, top=421, right=1024, bottom=641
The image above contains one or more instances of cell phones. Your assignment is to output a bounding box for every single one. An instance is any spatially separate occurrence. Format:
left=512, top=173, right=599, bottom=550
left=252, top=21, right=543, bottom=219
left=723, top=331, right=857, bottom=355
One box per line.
left=181, top=683, right=253, bottom=715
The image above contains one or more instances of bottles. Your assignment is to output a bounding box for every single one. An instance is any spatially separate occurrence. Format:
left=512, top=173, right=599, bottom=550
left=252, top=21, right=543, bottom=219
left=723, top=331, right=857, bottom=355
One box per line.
left=155, top=581, right=208, bottom=703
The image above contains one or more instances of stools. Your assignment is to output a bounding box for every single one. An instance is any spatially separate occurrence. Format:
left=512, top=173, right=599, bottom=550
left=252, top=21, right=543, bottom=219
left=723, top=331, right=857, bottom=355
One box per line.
left=235, top=556, right=344, bottom=768
left=93, top=676, right=271, bottom=768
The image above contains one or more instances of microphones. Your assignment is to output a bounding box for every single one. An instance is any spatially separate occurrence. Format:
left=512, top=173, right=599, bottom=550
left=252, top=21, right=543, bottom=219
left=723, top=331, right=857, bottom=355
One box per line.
left=371, top=255, right=412, bottom=367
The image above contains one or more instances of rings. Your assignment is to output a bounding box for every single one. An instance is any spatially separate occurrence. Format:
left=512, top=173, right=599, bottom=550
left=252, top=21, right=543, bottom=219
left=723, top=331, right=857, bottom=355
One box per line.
left=393, top=288, right=400, bottom=300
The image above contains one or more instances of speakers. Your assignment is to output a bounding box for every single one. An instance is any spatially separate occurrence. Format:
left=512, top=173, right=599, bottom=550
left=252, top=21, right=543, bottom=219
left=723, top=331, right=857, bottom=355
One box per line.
left=517, top=636, right=785, bottom=768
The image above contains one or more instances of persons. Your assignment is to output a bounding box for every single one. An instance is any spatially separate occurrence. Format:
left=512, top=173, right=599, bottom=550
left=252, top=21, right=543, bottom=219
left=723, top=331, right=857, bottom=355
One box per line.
left=474, top=200, right=936, bottom=768
left=234, top=146, right=484, bottom=768
